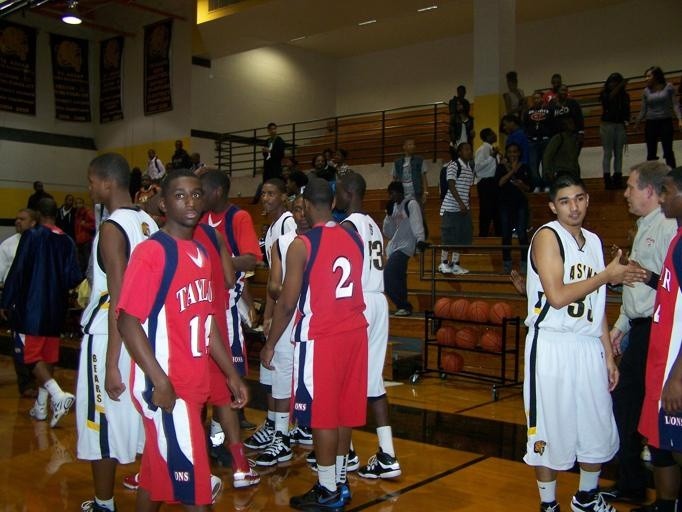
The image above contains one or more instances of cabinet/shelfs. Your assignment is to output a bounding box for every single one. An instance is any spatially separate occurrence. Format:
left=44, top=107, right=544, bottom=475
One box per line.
left=408, top=308, right=523, bottom=401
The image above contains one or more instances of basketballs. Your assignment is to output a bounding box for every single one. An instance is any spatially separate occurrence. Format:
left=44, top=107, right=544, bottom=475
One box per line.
left=441, top=352, right=464, bottom=372
left=434, top=297, right=512, bottom=352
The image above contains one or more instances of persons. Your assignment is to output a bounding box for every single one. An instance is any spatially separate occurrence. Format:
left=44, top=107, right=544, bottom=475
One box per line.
left=520, top=175, right=647, bottom=511
left=437, top=65, right=680, bottom=299
left=0, top=137, right=429, bottom=511
left=622, top=166, right=682, bottom=512
left=247, top=122, right=286, bottom=206
left=599, top=159, right=678, bottom=506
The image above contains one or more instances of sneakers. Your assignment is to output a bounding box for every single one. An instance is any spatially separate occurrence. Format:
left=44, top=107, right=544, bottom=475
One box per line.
left=21, top=388, right=76, bottom=428
left=438, top=260, right=470, bottom=274
left=80, top=472, right=139, bottom=512
left=394, top=308, right=411, bottom=316
left=541, top=486, right=682, bottom=512
left=233, top=416, right=402, bottom=512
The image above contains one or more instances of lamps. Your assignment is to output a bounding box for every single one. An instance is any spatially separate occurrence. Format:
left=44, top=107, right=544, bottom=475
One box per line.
left=60, top=0, right=83, bottom=25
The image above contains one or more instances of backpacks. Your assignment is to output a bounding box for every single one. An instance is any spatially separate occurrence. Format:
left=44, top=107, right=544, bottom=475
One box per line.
left=440, top=159, right=461, bottom=200
